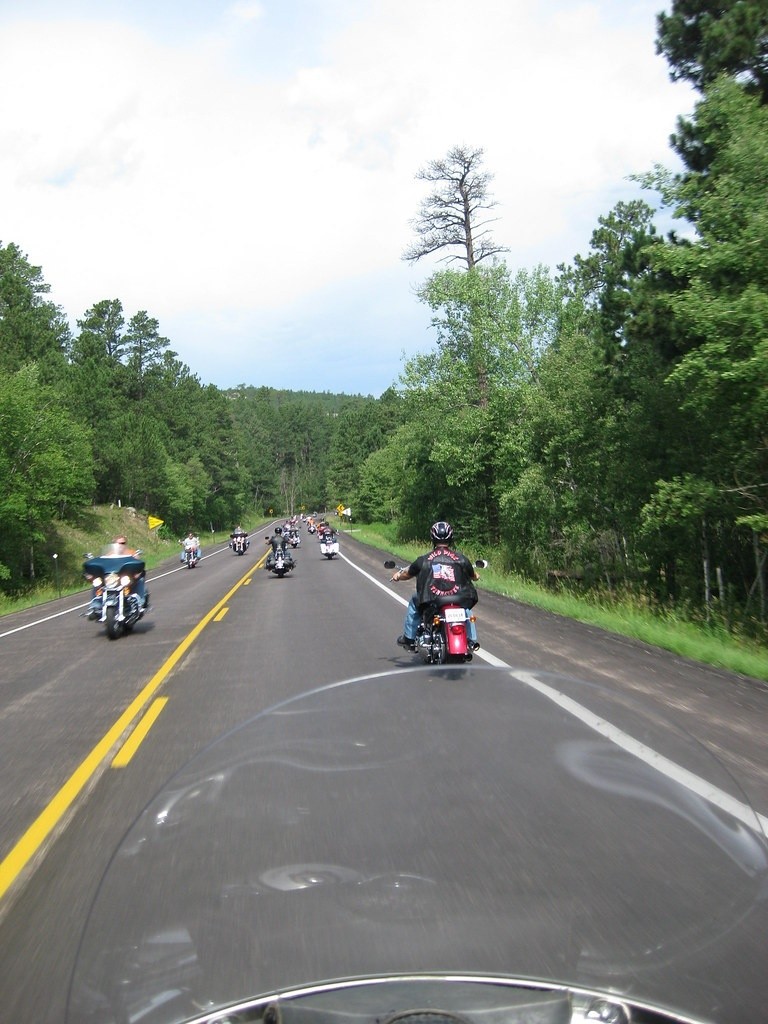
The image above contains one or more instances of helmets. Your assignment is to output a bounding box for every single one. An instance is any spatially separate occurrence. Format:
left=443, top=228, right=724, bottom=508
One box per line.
left=275, top=527, right=282, bottom=533
left=321, top=518, right=324, bottom=521
left=430, top=522, right=454, bottom=544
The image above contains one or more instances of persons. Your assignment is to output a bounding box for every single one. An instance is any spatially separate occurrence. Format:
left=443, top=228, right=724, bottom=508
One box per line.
left=233, top=511, right=325, bottom=542
left=268, top=527, right=291, bottom=559
left=180, top=532, right=201, bottom=562
left=393, top=523, right=477, bottom=649
left=320, top=521, right=339, bottom=542
left=89, top=536, right=145, bottom=620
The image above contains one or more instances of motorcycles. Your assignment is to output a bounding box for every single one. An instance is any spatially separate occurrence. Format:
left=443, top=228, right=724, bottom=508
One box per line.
left=264, top=535, right=297, bottom=577
left=79, top=549, right=152, bottom=639
left=316, top=524, right=327, bottom=539
left=305, top=521, right=317, bottom=534
left=381, top=559, right=489, bottom=679
left=316, top=530, right=340, bottom=559
left=282, top=519, right=302, bottom=548
left=301, top=511, right=317, bottom=523
left=177, top=538, right=200, bottom=568
left=230, top=527, right=250, bottom=555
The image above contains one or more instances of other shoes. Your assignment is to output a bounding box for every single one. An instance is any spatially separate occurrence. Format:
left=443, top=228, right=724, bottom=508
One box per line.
left=196, top=557, right=200, bottom=560
left=180, top=559, right=185, bottom=563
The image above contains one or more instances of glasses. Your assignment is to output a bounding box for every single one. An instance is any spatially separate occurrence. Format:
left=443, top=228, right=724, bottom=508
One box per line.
left=115, top=542, right=125, bottom=545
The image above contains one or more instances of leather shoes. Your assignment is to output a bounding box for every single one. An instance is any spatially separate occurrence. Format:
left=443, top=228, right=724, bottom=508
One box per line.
left=397, top=636, right=416, bottom=650
